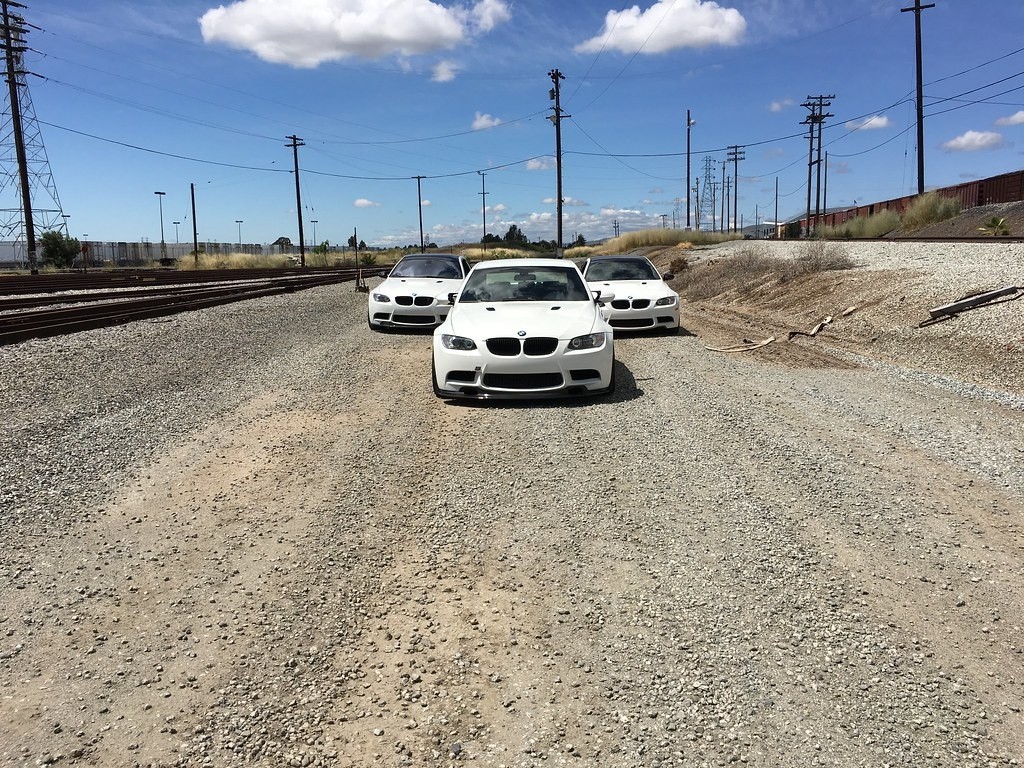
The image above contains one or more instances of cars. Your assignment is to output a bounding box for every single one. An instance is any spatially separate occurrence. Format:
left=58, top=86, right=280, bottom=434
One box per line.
left=430, top=258, right=615, bottom=401
left=579, top=255, right=680, bottom=334
left=368, top=254, right=473, bottom=331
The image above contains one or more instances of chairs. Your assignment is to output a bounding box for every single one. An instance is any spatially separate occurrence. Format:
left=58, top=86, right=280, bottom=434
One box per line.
left=543, top=281, right=560, bottom=299
left=494, top=281, right=511, bottom=300
